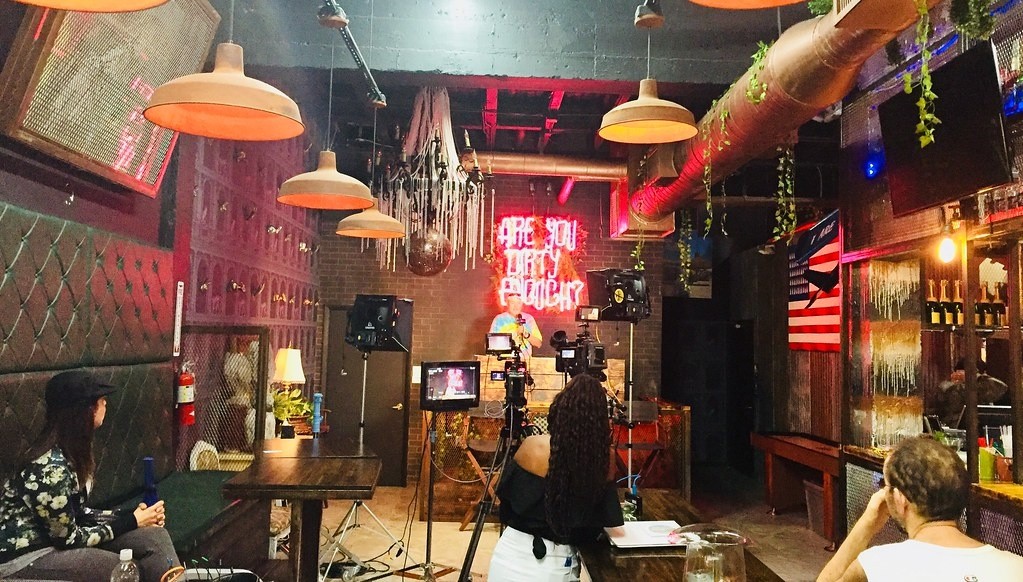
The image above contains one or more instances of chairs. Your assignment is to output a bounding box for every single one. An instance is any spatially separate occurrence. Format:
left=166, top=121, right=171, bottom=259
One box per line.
left=615, top=399, right=670, bottom=490
left=459, top=399, right=508, bottom=532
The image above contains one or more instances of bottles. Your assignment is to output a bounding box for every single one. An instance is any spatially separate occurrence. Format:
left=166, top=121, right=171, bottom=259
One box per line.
left=141, top=458, right=159, bottom=508
left=924, top=297, right=1007, bottom=328
left=110, top=549, right=140, bottom=582
left=668, top=523, right=751, bottom=582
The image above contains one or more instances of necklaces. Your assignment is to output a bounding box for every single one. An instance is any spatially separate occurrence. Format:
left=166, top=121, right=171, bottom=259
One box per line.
left=912, top=525, right=957, bottom=539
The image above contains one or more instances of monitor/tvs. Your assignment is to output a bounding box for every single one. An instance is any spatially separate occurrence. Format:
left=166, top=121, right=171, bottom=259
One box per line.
left=878, top=38, right=1013, bottom=219
left=420, top=360, right=480, bottom=410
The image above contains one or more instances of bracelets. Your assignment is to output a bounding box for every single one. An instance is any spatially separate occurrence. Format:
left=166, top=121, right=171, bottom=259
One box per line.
left=524, top=334, right=530, bottom=339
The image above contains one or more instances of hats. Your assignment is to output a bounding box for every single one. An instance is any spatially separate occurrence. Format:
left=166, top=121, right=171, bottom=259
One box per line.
left=45, top=371, right=117, bottom=414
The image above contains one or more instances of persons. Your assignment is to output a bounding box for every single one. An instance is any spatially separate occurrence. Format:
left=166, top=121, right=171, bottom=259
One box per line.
left=219, top=385, right=256, bottom=451
left=490, top=292, right=543, bottom=356
left=487, top=375, right=623, bottom=582
left=816, top=436, right=1023, bottom=582
left=0, top=371, right=183, bottom=582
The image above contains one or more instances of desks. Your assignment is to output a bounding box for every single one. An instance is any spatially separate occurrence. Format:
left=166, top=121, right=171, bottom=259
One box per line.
left=222, top=457, right=383, bottom=582
left=749, top=429, right=844, bottom=542
left=573, top=489, right=784, bottom=582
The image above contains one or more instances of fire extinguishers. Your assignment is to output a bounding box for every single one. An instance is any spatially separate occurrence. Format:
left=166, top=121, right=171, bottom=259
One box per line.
left=178, top=360, right=198, bottom=427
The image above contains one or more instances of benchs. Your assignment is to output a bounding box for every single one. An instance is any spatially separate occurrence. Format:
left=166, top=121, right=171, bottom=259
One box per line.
left=108, top=468, right=271, bottom=582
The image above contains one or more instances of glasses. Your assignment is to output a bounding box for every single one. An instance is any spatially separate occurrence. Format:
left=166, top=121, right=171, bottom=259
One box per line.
left=877, top=478, right=910, bottom=502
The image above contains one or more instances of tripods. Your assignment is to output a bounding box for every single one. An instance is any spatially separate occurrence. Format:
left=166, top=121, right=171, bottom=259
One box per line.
left=457, top=400, right=532, bottom=582
left=319, top=350, right=418, bottom=582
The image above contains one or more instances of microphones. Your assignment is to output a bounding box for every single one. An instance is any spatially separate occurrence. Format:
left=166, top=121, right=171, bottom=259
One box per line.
left=517, top=313, right=526, bottom=327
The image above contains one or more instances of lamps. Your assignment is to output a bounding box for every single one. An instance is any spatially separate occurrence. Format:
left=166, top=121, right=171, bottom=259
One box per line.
left=598, top=0, right=802, bottom=144
left=12, top=0, right=406, bottom=239
left=274, top=347, right=306, bottom=400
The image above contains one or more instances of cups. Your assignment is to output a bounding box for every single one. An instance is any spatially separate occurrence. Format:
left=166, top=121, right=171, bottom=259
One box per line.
left=937, top=429, right=998, bottom=481
